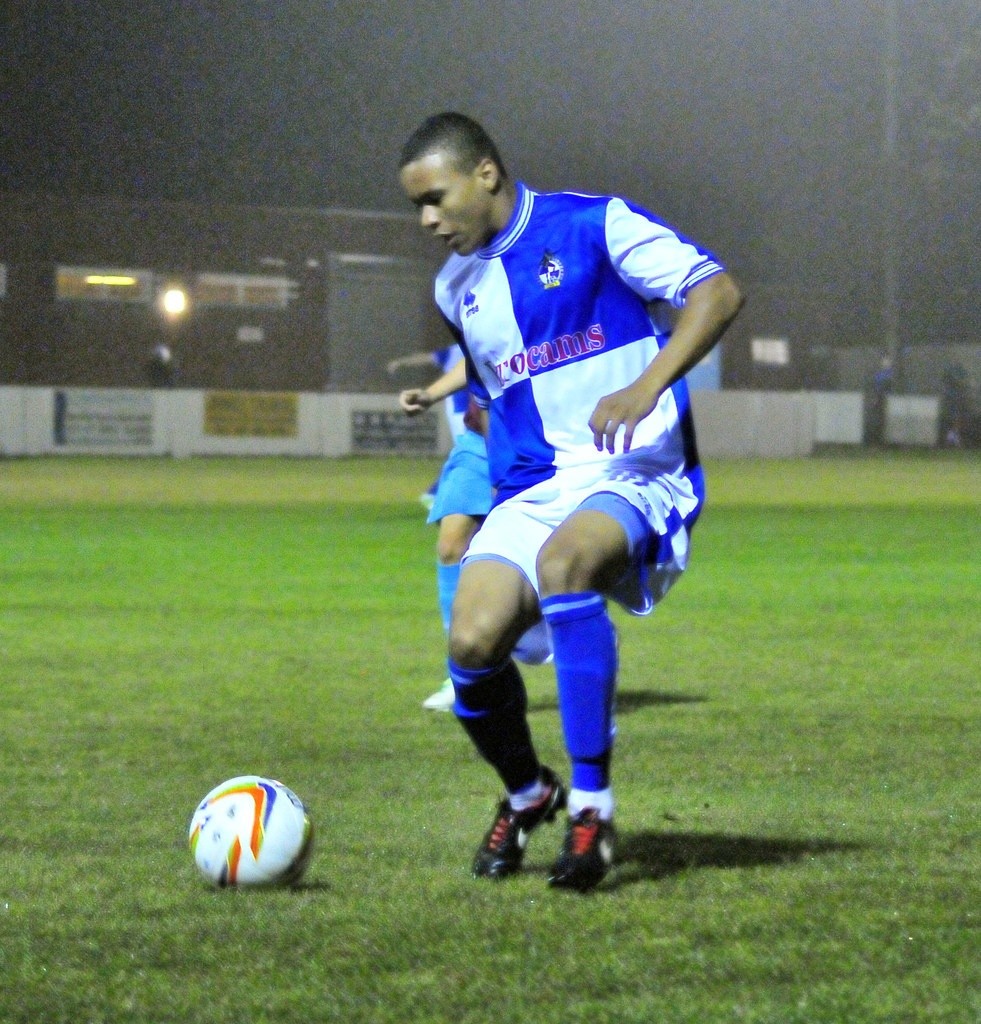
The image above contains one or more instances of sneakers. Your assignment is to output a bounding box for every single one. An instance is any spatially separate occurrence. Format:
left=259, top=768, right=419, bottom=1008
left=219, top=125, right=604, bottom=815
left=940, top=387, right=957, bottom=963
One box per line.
left=471, top=766, right=567, bottom=881
left=546, top=807, right=617, bottom=893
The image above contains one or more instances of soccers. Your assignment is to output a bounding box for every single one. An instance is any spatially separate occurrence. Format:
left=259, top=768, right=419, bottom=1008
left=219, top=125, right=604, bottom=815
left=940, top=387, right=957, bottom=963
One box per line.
left=185, top=771, right=316, bottom=892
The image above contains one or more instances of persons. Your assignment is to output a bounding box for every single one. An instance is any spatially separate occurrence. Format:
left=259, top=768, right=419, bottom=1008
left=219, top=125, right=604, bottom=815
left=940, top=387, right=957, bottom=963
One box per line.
left=400, top=338, right=488, bottom=711
left=399, top=112, right=742, bottom=893
left=933, top=351, right=981, bottom=451
left=863, top=355, right=895, bottom=454
left=143, top=347, right=176, bottom=389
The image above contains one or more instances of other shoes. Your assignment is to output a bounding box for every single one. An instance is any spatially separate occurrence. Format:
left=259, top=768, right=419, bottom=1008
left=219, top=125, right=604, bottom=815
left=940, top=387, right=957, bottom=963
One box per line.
left=423, top=677, right=456, bottom=712
